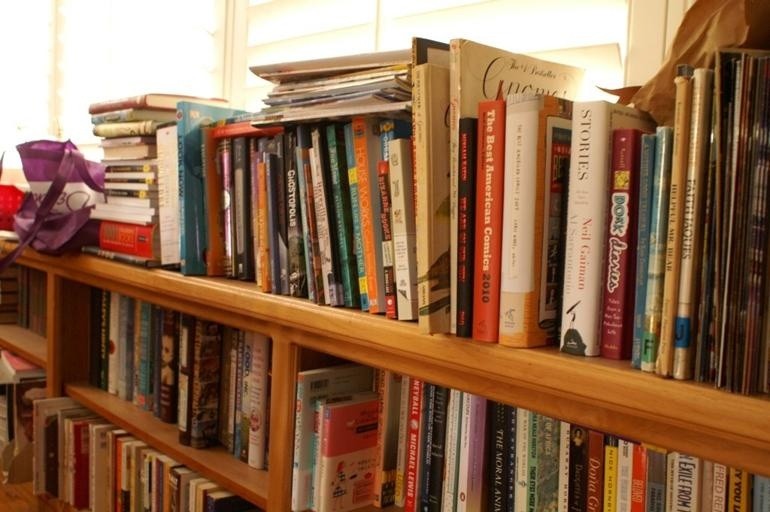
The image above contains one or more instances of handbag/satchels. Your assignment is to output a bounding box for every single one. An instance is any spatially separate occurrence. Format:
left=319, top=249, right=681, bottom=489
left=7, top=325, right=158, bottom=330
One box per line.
left=12, top=138, right=103, bottom=256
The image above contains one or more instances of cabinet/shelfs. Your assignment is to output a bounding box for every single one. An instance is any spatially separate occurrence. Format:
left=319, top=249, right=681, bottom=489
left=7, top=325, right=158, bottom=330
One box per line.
left=0, top=249, right=770, bottom=510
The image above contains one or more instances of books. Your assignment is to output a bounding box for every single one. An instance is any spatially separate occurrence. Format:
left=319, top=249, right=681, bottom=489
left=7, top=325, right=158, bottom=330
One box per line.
left=0, top=260, right=47, bottom=337
left=106, top=291, right=770, bottom=511
left=0, top=348, right=114, bottom=510
left=84, top=33, right=769, bottom=398
